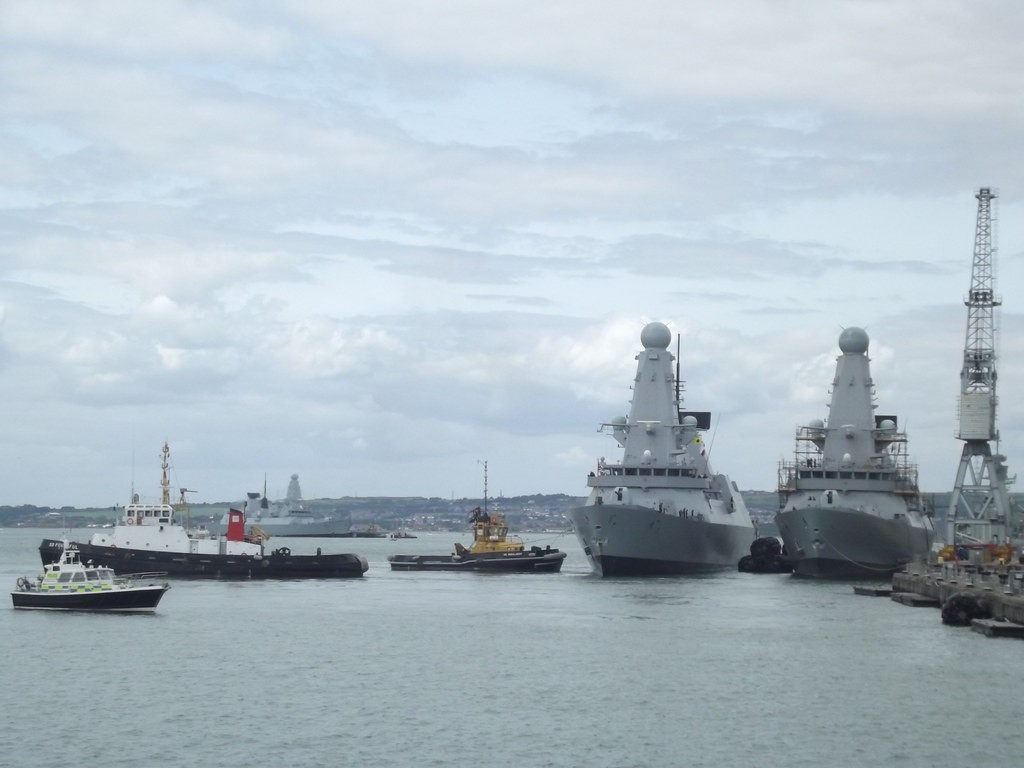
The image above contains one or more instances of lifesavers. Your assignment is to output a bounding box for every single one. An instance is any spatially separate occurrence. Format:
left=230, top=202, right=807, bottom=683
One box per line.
left=16, top=577, right=25, bottom=587
left=491, top=515, right=496, bottom=523
left=127, top=517, right=134, bottom=525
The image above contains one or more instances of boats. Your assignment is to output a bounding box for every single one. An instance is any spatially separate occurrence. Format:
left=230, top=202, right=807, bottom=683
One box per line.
left=388, top=460, right=567, bottom=573
left=573, top=323, right=754, bottom=574
left=391, top=531, right=417, bottom=539
left=219, top=473, right=349, bottom=536
left=773, top=326, right=935, bottom=578
left=356, top=522, right=387, bottom=538
left=10, top=517, right=171, bottom=612
left=39, top=441, right=369, bottom=578
